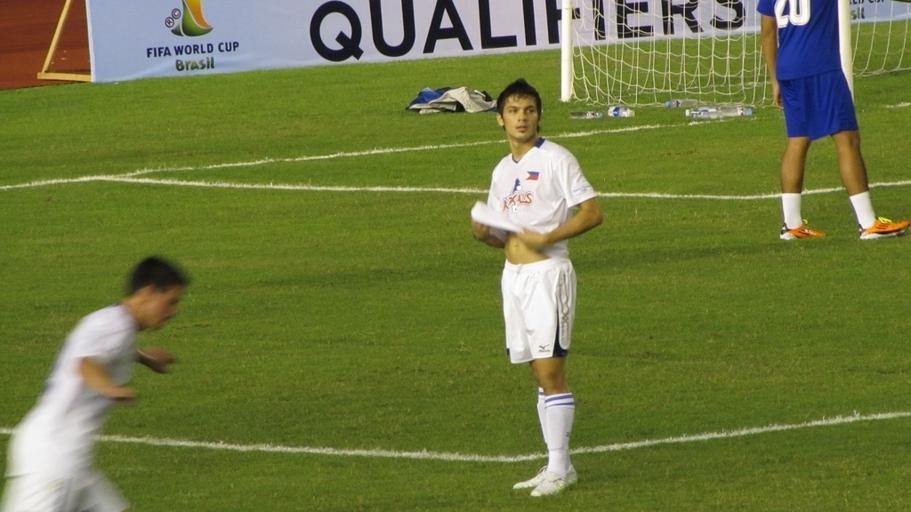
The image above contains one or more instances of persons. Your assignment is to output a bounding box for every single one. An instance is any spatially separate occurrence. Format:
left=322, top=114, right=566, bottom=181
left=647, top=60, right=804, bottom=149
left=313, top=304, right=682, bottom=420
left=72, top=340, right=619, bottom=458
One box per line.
left=467, top=78, right=605, bottom=500
left=0, top=253, right=193, bottom=511
left=756, top=1, right=911, bottom=242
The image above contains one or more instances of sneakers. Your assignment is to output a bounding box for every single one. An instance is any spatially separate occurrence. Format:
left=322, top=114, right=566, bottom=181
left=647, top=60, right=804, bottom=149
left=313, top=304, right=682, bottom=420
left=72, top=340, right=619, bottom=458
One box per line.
left=857, top=217, right=910, bottom=239
left=512, top=463, right=580, bottom=497
left=779, top=222, right=826, bottom=240
